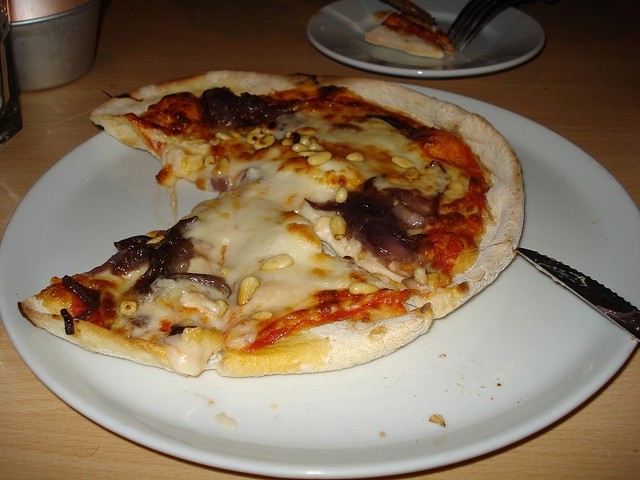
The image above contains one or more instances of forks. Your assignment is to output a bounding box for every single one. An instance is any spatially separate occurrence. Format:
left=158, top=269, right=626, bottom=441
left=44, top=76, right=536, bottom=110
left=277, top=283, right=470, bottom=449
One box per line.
left=450, top=1, right=509, bottom=50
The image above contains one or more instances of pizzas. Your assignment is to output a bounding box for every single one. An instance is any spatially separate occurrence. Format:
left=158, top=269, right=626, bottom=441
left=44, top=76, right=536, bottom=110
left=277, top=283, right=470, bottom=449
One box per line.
left=365, top=10, right=458, bottom=59
left=18, top=68, right=526, bottom=379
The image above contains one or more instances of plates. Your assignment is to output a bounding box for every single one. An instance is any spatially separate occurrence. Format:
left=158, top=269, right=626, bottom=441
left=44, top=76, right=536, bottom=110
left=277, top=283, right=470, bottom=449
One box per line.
left=306, top=0, right=545, bottom=78
left=0, top=77, right=640, bottom=480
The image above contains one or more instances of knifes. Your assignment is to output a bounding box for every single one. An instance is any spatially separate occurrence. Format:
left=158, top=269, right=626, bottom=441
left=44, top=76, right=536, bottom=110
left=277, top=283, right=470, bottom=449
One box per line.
left=515, top=247, right=640, bottom=350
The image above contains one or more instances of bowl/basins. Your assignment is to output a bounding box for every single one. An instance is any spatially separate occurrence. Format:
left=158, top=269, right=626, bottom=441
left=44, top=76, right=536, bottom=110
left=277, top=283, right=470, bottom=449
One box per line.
left=6, top=1, right=105, bottom=95
